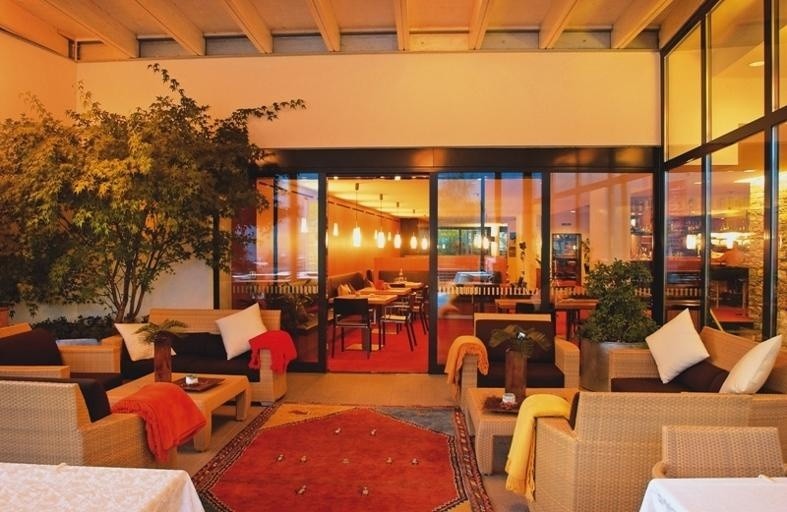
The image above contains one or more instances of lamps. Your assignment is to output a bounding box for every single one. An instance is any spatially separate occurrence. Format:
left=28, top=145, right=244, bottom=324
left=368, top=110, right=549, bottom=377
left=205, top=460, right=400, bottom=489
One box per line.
left=330, top=179, right=428, bottom=250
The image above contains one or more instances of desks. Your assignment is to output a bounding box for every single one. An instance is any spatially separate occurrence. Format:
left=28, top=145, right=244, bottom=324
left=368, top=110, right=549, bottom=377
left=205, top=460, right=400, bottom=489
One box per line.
left=453, top=271, right=493, bottom=282
left=0, top=461, right=205, bottom=512
left=494, top=295, right=600, bottom=341
left=329, top=279, right=423, bottom=350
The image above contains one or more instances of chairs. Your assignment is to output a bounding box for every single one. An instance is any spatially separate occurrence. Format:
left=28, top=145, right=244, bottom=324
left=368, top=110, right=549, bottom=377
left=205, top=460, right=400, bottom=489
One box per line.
left=0, top=323, right=123, bottom=412
left=332, top=285, right=429, bottom=358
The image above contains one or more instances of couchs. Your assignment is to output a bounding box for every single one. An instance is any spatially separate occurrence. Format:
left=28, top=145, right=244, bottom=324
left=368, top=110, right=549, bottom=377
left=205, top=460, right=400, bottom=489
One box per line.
left=0, top=375, right=179, bottom=468
left=100, top=307, right=289, bottom=406
left=289, top=269, right=368, bottom=357
left=379, top=267, right=431, bottom=299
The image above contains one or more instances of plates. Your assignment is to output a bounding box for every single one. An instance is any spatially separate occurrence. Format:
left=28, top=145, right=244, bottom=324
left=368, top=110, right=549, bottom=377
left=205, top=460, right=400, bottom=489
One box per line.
left=481, top=396, right=528, bottom=413
left=172, top=375, right=226, bottom=391
left=389, top=283, right=406, bottom=288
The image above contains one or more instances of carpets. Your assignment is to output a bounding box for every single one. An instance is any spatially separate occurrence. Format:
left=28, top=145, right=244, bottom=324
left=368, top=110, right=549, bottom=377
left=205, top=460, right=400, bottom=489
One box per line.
left=297, top=316, right=475, bottom=375
left=188, top=399, right=494, bottom=511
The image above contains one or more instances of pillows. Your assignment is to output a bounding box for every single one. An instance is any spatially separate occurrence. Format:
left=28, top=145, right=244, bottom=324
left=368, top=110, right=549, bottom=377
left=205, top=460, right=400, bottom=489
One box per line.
left=113, top=315, right=176, bottom=362
left=0, top=326, right=65, bottom=366
left=215, top=301, right=270, bottom=360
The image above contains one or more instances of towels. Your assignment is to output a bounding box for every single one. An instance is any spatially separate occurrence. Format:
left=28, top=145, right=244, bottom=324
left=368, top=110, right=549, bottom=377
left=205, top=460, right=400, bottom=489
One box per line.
left=248, top=328, right=298, bottom=377
left=111, top=381, right=207, bottom=465
left=445, top=335, right=490, bottom=401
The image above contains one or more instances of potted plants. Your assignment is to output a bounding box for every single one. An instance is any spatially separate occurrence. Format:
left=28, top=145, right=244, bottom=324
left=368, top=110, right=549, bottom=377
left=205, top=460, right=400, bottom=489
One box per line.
left=132, top=316, right=187, bottom=383
